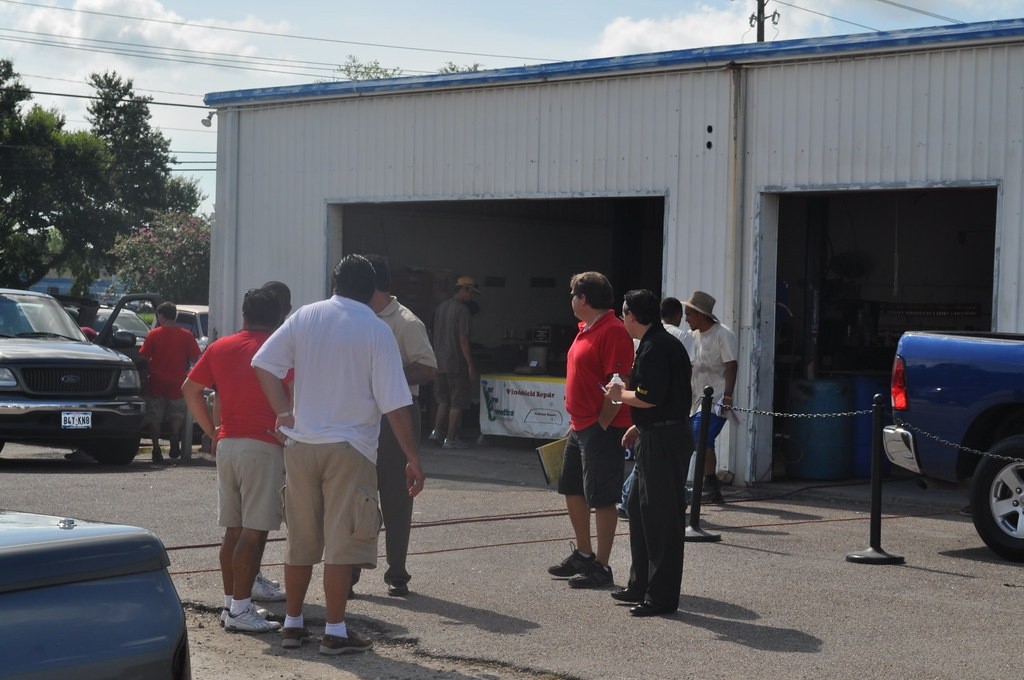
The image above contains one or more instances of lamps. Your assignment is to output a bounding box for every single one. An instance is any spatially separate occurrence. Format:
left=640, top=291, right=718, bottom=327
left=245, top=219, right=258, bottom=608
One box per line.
left=201, top=111, right=218, bottom=127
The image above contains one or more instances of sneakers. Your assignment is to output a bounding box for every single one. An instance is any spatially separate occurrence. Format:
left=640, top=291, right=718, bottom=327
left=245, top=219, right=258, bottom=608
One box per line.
left=611, top=588, right=644, bottom=601
left=282, top=622, right=307, bottom=647
left=220, top=607, right=269, bottom=625
left=225, top=606, right=281, bottom=635
left=630, top=600, right=676, bottom=615
left=568, top=561, right=614, bottom=588
left=250, top=571, right=286, bottom=602
left=319, top=625, right=374, bottom=655
left=548, top=541, right=596, bottom=576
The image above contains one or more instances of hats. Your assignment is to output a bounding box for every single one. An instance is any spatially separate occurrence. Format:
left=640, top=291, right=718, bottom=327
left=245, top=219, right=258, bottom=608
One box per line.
left=680, top=290, right=720, bottom=322
left=455, top=275, right=484, bottom=295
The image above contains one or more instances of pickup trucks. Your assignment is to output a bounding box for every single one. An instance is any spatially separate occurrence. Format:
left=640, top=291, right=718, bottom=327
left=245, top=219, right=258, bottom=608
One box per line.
left=881, top=329, right=1024, bottom=569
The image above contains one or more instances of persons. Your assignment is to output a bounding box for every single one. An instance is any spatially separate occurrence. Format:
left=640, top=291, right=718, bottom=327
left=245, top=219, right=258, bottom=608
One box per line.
left=63, top=327, right=98, bottom=461
left=211, top=280, right=292, bottom=599
left=426, top=276, right=482, bottom=450
left=347, top=254, right=438, bottom=598
left=182, top=290, right=280, bottom=634
left=546, top=272, right=697, bottom=616
left=138, top=303, right=203, bottom=463
left=680, top=290, right=737, bottom=504
left=250, top=254, right=425, bottom=655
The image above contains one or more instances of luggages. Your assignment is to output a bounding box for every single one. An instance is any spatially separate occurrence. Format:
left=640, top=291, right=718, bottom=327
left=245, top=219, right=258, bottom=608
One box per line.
left=688, top=479, right=725, bottom=504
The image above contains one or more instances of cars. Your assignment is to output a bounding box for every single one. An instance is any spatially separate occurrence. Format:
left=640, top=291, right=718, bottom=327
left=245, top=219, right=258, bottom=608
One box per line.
left=63, top=301, right=151, bottom=353
left=0, top=507, right=193, bottom=680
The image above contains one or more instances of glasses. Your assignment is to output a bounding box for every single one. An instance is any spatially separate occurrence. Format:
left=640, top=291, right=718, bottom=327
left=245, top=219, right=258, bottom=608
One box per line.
left=568, top=293, right=576, bottom=301
left=619, top=314, right=624, bottom=320
left=244, top=288, right=254, bottom=307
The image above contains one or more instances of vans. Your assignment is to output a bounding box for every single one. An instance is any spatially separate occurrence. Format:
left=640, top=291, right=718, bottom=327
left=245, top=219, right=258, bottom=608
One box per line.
left=151, top=304, right=210, bottom=353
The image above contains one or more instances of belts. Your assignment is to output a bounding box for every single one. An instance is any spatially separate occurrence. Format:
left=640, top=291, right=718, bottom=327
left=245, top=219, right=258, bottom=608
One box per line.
left=640, top=419, right=688, bottom=431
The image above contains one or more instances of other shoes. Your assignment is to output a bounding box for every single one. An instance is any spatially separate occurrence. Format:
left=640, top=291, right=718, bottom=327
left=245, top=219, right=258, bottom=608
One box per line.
left=429, top=427, right=447, bottom=444
left=347, top=586, right=355, bottom=598
left=442, top=433, right=468, bottom=449
left=387, top=581, right=408, bottom=596
left=616, top=504, right=628, bottom=518
left=152, top=450, right=163, bottom=464
left=169, top=435, right=181, bottom=458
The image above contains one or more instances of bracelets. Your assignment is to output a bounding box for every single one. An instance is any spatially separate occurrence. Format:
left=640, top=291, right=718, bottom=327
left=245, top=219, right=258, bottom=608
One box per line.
left=277, top=410, right=292, bottom=417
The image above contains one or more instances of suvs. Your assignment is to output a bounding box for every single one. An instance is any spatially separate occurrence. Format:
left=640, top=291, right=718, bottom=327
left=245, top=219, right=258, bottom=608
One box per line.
left=1, top=287, right=148, bottom=470
